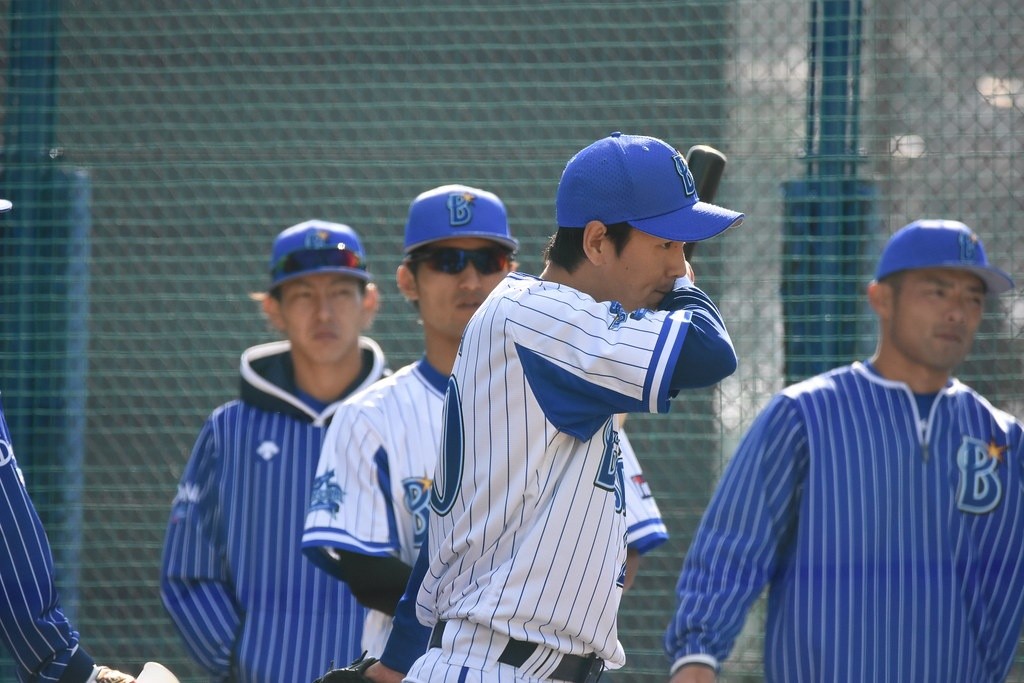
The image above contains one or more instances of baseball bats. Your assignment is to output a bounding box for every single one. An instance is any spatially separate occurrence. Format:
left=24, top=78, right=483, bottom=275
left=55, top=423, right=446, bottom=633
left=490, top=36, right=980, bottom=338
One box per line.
left=682, top=139, right=728, bottom=264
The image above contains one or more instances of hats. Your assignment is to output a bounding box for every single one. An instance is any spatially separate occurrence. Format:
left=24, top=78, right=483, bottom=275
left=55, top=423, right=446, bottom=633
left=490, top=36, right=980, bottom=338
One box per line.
left=268, top=220, right=373, bottom=298
left=875, top=217, right=1016, bottom=293
left=403, top=183, right=520, bottom=256
left=555, top=130, right=746, bottom=242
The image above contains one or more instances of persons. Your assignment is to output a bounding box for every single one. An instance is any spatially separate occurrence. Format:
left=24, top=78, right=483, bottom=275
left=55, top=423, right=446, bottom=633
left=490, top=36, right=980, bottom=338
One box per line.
left=161, top=219, right=392, bottom=683
left=301, top=183, right=669, bottom=660
left=664, top=219, right=1024, bottom=683
left=0, top=397, right=134, bottom=683
left=312, top=132, right=744, bottom=683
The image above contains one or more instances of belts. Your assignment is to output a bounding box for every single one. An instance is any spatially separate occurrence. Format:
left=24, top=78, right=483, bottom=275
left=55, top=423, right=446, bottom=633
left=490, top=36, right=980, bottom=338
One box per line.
left=426, top=621, right=605, bottom=682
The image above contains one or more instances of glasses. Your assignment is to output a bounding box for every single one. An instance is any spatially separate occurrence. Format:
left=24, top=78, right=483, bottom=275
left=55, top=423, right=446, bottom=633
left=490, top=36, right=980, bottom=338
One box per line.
left=409, top=245, right=507, bottom=275
left=282, top=246, right=365, bottom=273
